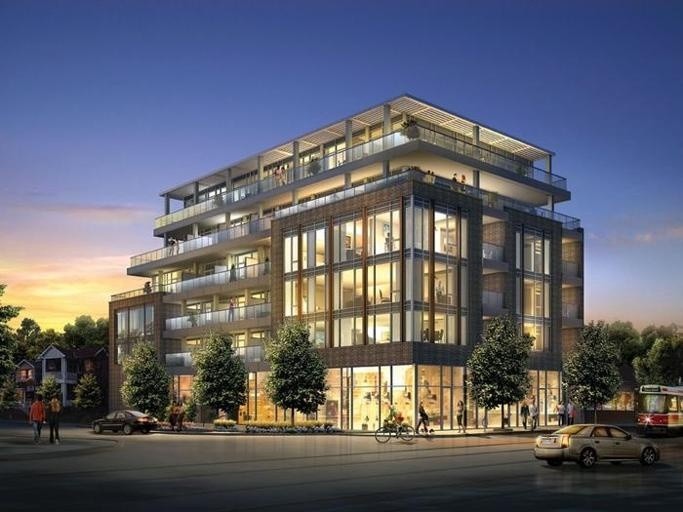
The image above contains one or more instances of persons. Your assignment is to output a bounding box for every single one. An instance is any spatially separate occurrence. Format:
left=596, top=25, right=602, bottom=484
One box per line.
left=227, top=294, right=237, bottom=323
left=176, top=400, right=185, bottom=431
left=169, top=400, right=179, bottom=432
left=528, top=399, right=539, bottom=429
left=186, top=311, right=197, bottom=327
left=413, top=401, right=431, bottom=436
left=278, top=167, right=287, bottom=187
left=451, top=172, right=457, bottom=182
left=272, top=164, right=281, bottom=187
left=554, top=400, right=566, bottom=427
left=435, top=279, right=445, bottom=303
left=518, top=400, right=530, bottom=430
left=166, top=237, right=177, bottom=256
left=456, top=400, right=466, bottom=433
left=228, top=263, right=237, bottom=283
left=262, top=257, right=270, bottom=275
left=28, top=393, right=45, bottom=445
left=566, top=397, right=576, bottom=424
left=46, top=392, right=62, bottom=443
left=384, top=404, right=407, bottom=438
left=459, top=175, right=466, bottom=185
